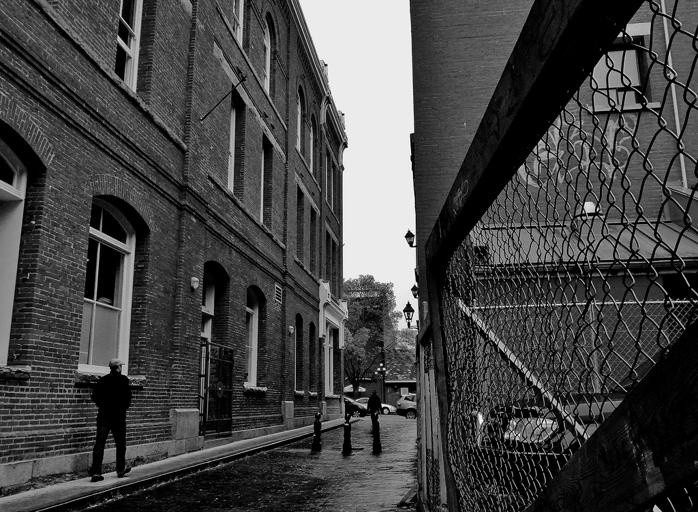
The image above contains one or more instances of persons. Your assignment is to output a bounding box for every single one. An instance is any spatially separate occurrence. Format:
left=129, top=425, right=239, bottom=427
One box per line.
left=91, top=359, right=132, bottom=482
left=368, top=390, right=381, bottom=435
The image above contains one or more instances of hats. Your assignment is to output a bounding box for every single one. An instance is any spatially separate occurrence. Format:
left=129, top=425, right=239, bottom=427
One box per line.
left=109, top=358, right=126, bottom=368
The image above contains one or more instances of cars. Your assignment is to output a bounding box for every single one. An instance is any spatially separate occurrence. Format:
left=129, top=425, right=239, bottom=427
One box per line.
left=354, top=397, right=397, bottom=413
left=485, top=347, right=667, bottom=499
left=343, top=395, right=366, bottom=417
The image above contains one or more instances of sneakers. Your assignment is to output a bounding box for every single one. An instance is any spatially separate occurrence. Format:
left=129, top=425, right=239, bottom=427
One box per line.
left=117, top=465, right=132, bottom=478
left=90, top=475, right=105, bottom=482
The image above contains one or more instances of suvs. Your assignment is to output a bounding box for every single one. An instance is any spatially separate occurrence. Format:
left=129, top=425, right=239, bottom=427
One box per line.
left=397, top=393, right=418, bottom=419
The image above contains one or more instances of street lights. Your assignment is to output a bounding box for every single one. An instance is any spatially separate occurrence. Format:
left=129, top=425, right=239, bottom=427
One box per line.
left=374, top=363, right=389, bottom=402
